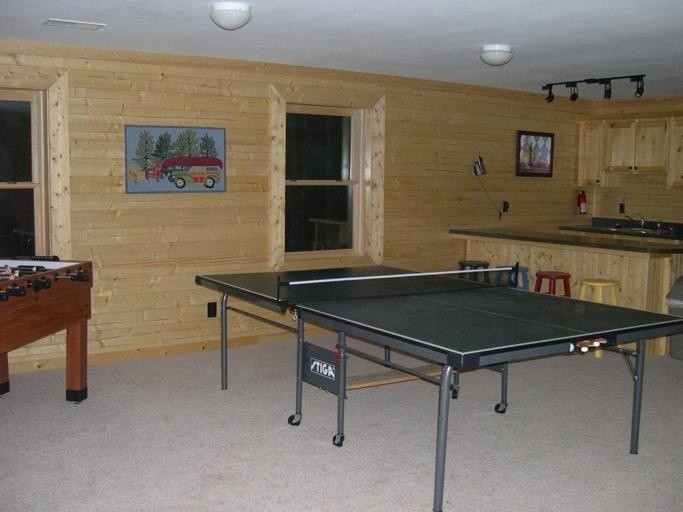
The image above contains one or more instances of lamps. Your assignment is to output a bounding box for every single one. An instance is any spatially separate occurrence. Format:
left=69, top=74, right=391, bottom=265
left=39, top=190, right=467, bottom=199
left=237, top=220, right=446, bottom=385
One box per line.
left=211, top=3, right=253, bottom=31
left=542, top=75, right=645, bottom=103
left=480, top=46, right=514, bottom=66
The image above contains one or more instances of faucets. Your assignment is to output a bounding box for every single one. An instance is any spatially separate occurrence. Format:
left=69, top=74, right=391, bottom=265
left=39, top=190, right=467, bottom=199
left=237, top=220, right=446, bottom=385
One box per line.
left=623, top=212, right=648, bottom=228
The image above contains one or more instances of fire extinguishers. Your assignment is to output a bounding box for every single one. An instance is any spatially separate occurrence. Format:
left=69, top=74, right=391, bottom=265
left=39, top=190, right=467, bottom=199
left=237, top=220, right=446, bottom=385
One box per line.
left=577, top=191, right=587, bottom=214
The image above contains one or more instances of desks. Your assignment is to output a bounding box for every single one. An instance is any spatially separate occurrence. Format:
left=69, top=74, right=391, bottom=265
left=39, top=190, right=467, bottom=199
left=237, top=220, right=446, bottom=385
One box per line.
left=195, top=265, right=683, bottom=512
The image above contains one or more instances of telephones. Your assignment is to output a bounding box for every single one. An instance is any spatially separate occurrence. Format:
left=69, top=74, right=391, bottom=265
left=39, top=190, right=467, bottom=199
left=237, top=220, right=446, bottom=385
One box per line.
left=474, top=155, right=486, bottom=175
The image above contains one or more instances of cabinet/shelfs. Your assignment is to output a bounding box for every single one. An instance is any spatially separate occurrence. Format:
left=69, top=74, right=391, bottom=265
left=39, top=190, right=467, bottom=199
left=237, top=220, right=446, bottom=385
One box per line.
left=577, top=120, right=618, bottom=186
left=667, top=116, right=683, bottom=188
left=604, top=118, right=669, bottom=174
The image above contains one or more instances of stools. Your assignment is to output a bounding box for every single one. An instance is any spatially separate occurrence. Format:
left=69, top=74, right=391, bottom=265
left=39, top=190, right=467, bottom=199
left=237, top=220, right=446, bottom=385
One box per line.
left=458, top=260, right=617, bottom=358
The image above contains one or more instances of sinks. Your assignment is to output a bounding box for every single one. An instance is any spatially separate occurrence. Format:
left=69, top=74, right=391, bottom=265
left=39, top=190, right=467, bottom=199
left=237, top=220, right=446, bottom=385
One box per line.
left=611, top=227, right=662, bottom=238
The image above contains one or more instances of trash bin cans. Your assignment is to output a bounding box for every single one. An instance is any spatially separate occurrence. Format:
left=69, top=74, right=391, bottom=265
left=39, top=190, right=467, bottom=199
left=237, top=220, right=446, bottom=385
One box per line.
left=665, top=275, right=683, bottom=360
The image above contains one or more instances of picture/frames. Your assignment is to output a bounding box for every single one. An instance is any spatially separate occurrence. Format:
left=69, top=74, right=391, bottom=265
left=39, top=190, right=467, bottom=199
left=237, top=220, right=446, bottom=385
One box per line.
left=516, top=130, right=555, bottom=177
